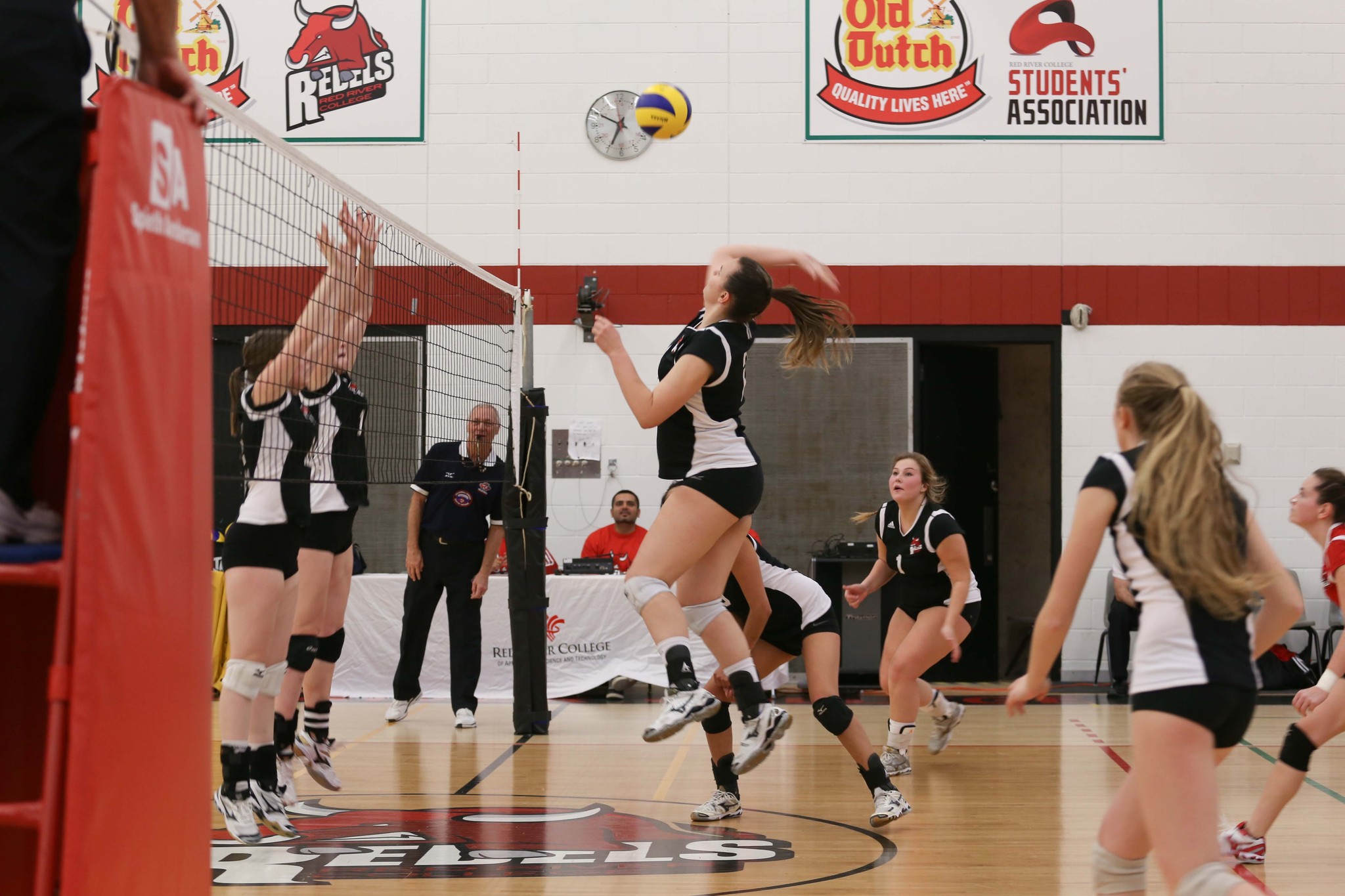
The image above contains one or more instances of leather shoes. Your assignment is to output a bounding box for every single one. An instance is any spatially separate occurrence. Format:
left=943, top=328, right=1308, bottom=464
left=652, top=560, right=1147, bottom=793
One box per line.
left=1107, top=679, right=1129, bottom=700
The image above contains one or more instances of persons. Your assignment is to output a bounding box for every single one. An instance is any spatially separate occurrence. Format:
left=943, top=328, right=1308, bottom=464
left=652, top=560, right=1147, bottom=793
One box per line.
left=842, top=451, right=983, bottom=778
left=1005, top=361, right=1305, bottom=896
left=0, top=0, right=206, bottom=542
left=592, top=246, right=841, bottom=775
left=580, top=490, right=648, bottom=700
left=378, top=404, right=505, bottom=729
left=684, top=527, right=913, bottom=828
left=1219, top=466, right=1345, bottom=866
left=490, top=529, right=557, bottom=574
left=1105, top=540, right=1142, bottom=692
left=213, top=200, right=383, bottom=846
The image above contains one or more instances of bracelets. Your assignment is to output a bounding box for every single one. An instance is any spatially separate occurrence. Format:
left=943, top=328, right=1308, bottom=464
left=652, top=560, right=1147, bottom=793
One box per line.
left=1316, top=668, right=1341, bottom=694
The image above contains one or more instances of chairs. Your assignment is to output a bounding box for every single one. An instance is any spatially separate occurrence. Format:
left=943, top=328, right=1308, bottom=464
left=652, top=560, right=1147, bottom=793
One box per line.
left=1094, top=570, right=1116, bottom=683
left=1322, top=600, right=1345, bottom=668
left=1251, top=571, right=1325, bottom=684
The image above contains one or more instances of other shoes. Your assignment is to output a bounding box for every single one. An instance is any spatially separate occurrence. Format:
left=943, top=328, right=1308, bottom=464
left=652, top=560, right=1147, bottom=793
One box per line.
left=607, top=676, right=637, bottom=699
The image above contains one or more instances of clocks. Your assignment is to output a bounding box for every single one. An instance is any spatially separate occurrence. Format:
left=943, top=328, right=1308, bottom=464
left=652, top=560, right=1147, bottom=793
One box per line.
left=586, top=91, right=652, bottom=160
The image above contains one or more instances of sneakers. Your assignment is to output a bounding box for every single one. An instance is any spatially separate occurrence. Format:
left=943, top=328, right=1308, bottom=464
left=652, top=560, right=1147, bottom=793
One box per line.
left=690, top=785, right=743, bottom=822
left=293, top=729, right=342, bottom=791
left=642, top=678, right=721, bottom=742
left=384, top=691, right=422, bottom=722
left=731, top=702, right=793, bottom=774
left=927, top=702, right=965, bottom=755
left=879, top=745, right=912, bottom=777
left=214, top=783, right=262, bottom=844
left=454, top=708, right=476, bottom=728
left=869, top=781, right=912, bottom=827
left=275, top=745, right=308, bottom=804
left=250, top=779, right=297, bottom=838
left=1218, top=820, right=1266, bottom=863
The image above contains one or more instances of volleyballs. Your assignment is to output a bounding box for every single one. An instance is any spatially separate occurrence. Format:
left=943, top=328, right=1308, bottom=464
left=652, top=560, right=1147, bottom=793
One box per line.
left=635, top=83, right=692, bottom=138
